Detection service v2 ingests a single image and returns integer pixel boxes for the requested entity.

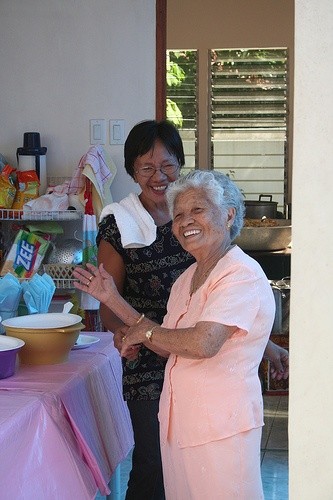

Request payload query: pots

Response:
[242,193,278,219]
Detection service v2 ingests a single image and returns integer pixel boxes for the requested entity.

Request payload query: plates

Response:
[2,313,82,329]
[72,334,101,350]
[0,335,25,351]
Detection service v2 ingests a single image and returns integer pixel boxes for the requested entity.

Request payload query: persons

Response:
[94,119,289,499]
[73,170,275,500]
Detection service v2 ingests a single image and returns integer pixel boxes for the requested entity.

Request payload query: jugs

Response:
[16,132,50,198]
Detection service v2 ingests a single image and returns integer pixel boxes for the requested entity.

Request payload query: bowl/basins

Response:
[230,219,292,252]
[0,346,25,381]
[5,323,86,367]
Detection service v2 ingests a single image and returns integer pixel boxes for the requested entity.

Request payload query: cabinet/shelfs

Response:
[1,168,106,333]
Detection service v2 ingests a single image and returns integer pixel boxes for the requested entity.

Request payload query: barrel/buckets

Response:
[268,276,291,337]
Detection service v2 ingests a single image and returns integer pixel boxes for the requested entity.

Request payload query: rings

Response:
[121,336,126,342]
[86,281,90,285]
[88,276,93,280]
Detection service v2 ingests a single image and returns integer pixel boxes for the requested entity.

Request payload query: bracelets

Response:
[136,313,144,324]
[145,326,159,345]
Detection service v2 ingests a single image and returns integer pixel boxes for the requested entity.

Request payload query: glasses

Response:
[136,163,180,175]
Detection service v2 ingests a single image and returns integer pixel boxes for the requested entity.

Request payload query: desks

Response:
[1,330,135,499]
